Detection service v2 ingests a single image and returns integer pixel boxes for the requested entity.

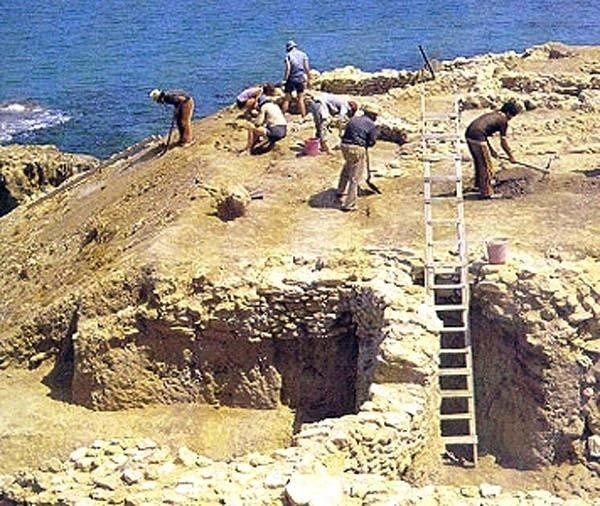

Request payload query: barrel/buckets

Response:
[486,237,508,264]
[305,137,321,156]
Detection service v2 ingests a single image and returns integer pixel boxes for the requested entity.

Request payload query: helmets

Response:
[363,103,379,114]
[347,100,357,112]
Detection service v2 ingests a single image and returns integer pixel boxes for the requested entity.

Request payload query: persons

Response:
[236,80,356,157]
[147,87,195,148]
[281,39,310,120]
[336,105,377,211]
[464,103,517,200]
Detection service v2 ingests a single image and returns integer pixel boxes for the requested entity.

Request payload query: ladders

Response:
[421,85,478,468]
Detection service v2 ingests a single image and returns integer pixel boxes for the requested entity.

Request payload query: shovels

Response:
[365,147,381,194]
[160,103,179,157]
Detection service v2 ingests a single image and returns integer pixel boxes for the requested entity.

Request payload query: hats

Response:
[257,94,268,106]
[284,40,297,52]
[149,88,164,103]
